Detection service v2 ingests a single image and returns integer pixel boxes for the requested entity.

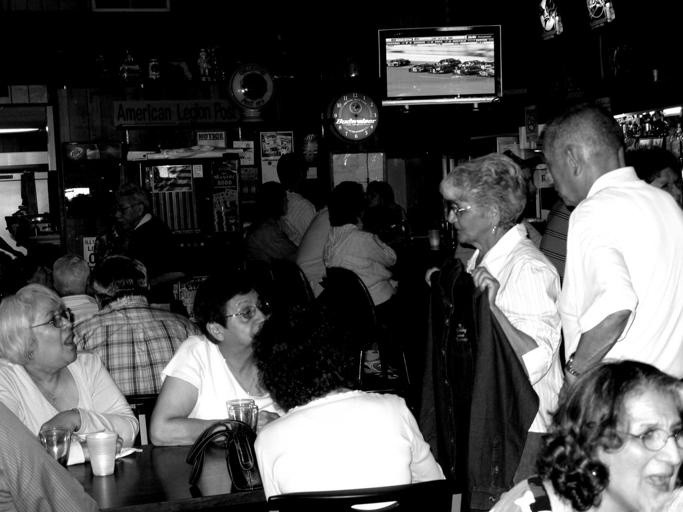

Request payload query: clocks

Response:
[329,89,382,141]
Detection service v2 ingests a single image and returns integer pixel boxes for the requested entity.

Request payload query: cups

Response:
[86,430,117,476]
[38,426,71,469]
[226,398,258,432]
[441,220,456,249]
[428,229,440,248]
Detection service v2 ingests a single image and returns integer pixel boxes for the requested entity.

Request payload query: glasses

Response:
[601,421,682,453]
[449,204,482,218]
[28,307,75,329]
[211,299,263,322]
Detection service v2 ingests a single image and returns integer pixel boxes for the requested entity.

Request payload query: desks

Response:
[50,432,267,511]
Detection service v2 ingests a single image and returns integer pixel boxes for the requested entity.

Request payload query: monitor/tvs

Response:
[377,24,504,107]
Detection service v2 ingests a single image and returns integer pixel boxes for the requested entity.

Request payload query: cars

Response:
[409,58,495,77]
[386,59,411,68]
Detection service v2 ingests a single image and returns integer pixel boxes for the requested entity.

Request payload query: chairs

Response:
[116,394,160,448]
[254,478,458,511]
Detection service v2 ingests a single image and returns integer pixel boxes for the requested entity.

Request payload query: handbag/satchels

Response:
[186,419,264,496]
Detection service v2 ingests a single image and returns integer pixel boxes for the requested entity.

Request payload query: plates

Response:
[166,144,214,156]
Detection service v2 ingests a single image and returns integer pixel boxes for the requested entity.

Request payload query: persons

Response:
[0,108,682,512]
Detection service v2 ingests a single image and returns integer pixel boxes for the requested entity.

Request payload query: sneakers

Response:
[364,359,401,381]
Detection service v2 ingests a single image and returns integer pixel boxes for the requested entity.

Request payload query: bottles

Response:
[616,109,683,158]
